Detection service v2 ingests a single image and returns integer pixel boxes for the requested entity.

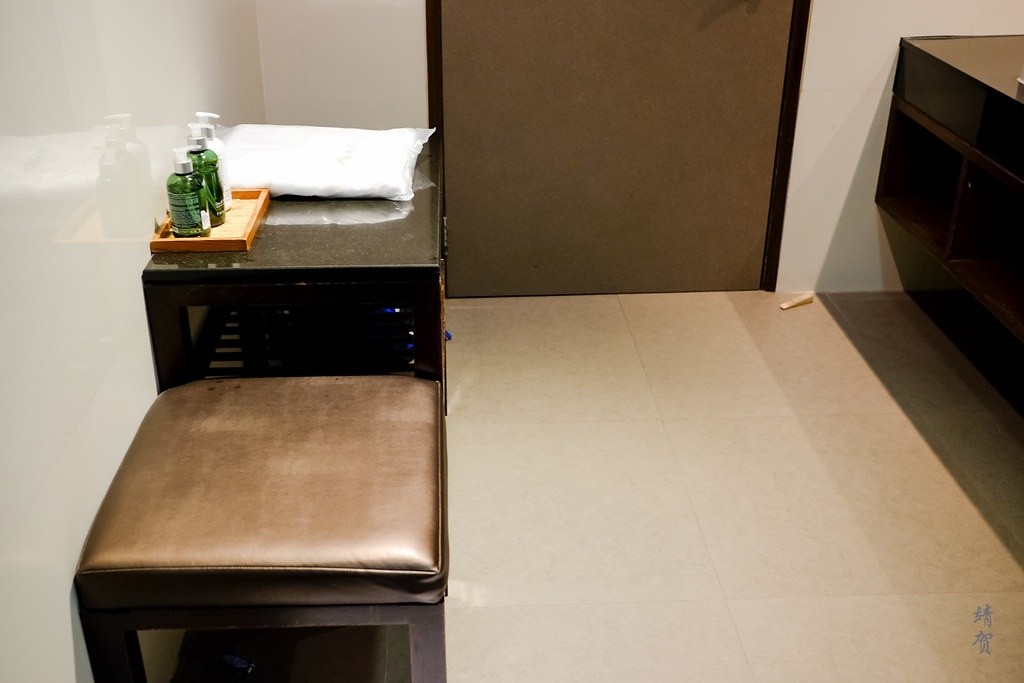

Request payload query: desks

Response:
[141,129,448,416]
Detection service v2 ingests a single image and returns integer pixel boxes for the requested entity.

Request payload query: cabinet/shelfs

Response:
[874,34,1024,420]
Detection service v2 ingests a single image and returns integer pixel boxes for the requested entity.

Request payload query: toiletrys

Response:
[165,111,233,238]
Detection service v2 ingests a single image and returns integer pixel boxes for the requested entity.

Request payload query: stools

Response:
[73,375,449,683]
